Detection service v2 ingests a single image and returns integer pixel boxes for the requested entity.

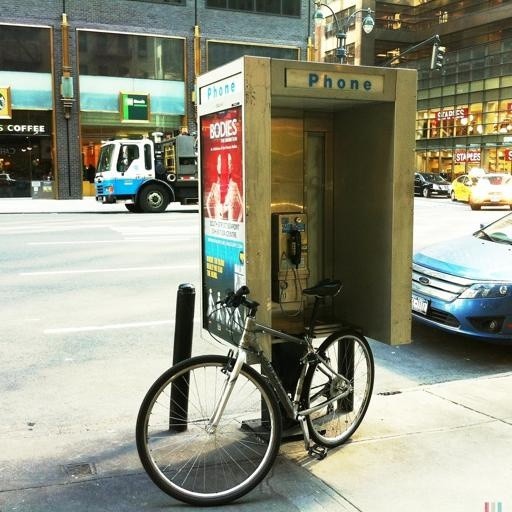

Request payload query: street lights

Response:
[313,3,376,65]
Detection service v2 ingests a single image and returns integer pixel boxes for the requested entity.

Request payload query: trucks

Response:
[92,134,197,214]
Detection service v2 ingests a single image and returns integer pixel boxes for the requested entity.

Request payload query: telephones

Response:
[271,211,311,281]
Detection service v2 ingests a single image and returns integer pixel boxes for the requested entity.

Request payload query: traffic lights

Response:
[430,43,447,73]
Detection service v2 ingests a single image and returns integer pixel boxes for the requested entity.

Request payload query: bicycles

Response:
[135,282,375,502]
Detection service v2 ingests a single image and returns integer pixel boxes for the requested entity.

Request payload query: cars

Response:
[414,166,512,213]
[410,201,512,359]
